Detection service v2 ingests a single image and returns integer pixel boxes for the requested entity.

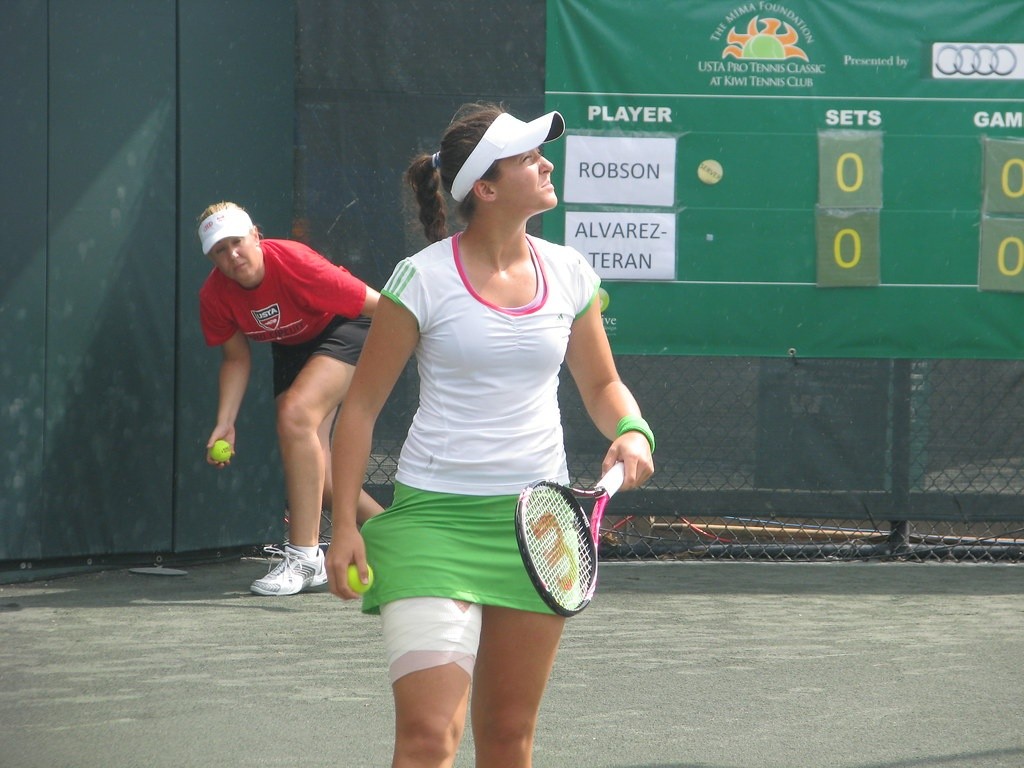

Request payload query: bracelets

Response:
[617,415,656,453]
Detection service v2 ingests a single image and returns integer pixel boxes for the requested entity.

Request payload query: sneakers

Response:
[250,543,328,595]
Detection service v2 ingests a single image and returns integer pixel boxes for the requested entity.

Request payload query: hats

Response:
[198,209,252,255]
[450,111,566,203]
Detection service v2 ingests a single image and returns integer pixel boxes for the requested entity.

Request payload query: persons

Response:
[324,104,655,768]
[198,200,387,598]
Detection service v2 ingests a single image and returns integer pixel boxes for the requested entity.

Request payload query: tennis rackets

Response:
[513,459,625,618]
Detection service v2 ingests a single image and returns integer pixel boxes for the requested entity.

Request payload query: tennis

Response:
[347,563,374,594]
[210,439,232,462]
